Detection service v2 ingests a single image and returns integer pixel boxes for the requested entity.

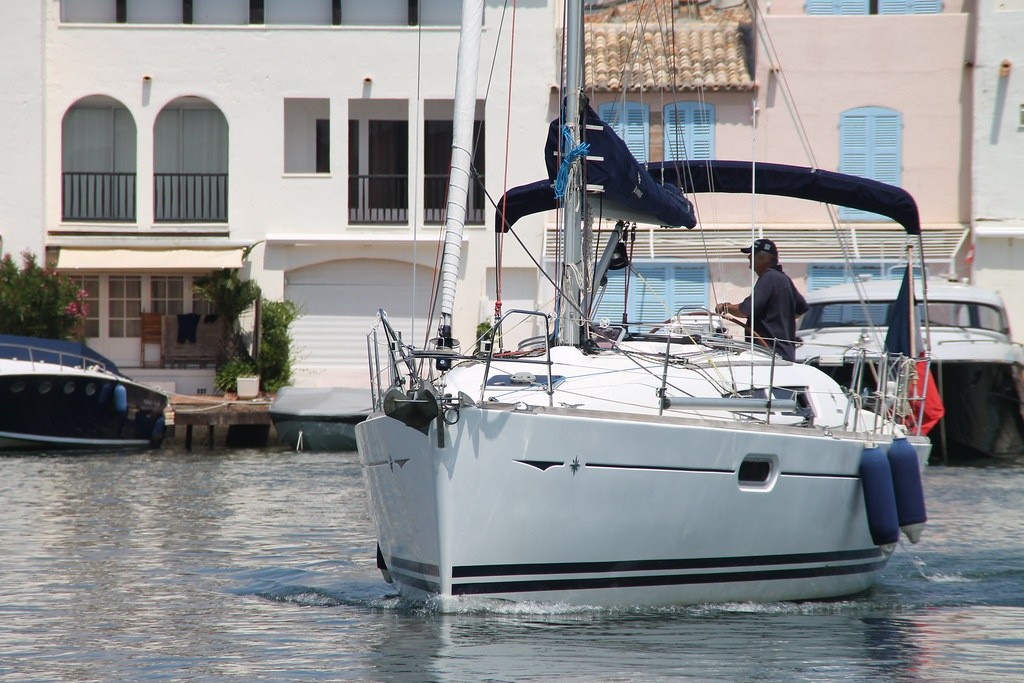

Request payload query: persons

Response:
[714,238,807,361]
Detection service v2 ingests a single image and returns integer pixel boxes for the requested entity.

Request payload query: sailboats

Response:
[353,0,944,613]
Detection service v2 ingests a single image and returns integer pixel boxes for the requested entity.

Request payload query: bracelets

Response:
[721,303,729,311]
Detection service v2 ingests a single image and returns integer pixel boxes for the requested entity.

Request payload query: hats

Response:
[741,238,778,254]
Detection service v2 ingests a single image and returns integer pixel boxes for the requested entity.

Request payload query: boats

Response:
[793,223,1024,463]
[267,385,386,452]
[0,334,168,452]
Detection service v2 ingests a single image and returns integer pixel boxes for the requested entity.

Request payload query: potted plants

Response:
[212,357,261,399]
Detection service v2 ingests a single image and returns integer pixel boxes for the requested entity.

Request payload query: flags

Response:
[884,263,943,436]
